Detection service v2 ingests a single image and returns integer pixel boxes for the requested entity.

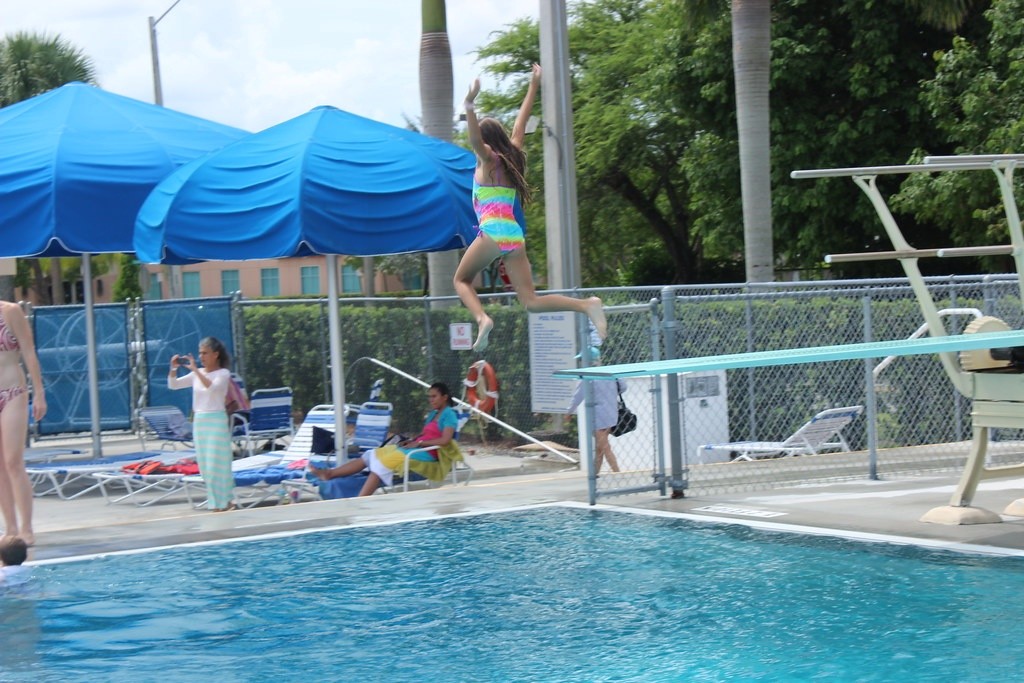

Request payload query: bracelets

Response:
[170,367,177,371]
[419,440,423,447]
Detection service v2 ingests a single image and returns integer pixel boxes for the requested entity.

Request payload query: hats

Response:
[574,346,600,359]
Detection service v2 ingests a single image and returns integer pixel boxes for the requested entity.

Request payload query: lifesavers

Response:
[462,358,501,416]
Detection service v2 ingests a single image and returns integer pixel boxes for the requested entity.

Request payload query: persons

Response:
[166,338,234,512]
[0,535,34,586]
[310,382,458,497]
[566,348,627,477]
[0,297,47,545]
[452,62,607,354]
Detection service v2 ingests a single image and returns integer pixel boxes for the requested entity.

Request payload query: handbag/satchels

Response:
[609,409,636,437]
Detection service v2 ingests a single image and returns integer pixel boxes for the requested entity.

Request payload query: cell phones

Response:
[177,358,190,365]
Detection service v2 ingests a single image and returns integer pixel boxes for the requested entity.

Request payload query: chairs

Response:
[137,406,194,450]
[91,450,286,507]
[229,387,296,456]
[280,406,475,505]
[22,447,89,483]
[695,405,864,465]
[228,402,393,512]
[181,405,350,510]
[25,452,198,501]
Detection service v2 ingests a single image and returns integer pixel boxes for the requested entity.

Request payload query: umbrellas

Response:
[0,82,528,477]
